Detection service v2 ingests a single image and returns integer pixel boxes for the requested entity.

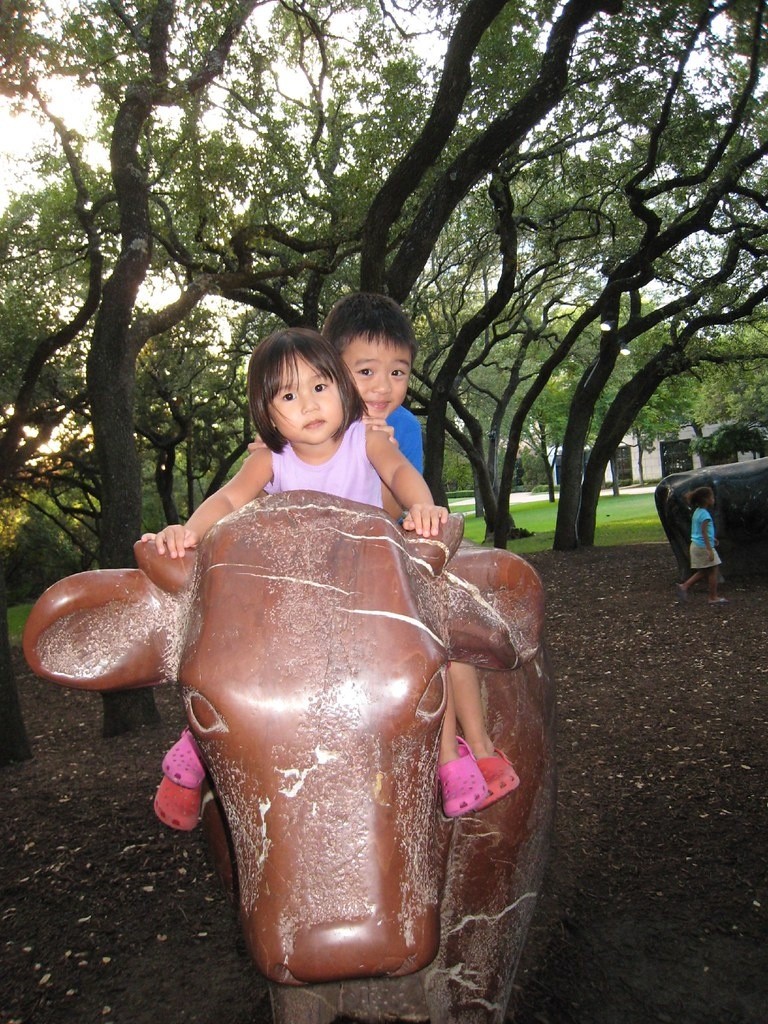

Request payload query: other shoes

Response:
[676,582,690,602]
[709,596,730,606]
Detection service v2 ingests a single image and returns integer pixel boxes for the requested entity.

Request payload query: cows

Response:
[653,455,768,592]
[21,488,560,1024]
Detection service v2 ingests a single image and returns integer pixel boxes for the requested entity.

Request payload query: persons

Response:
[154,292,519,830]
[676,487,728,605]
[141,328,486,815]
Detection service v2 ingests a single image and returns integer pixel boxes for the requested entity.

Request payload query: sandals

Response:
[162,722,207,789]
[470,746,518,812]
[154,775,202,832]
[437,734,488,817]
